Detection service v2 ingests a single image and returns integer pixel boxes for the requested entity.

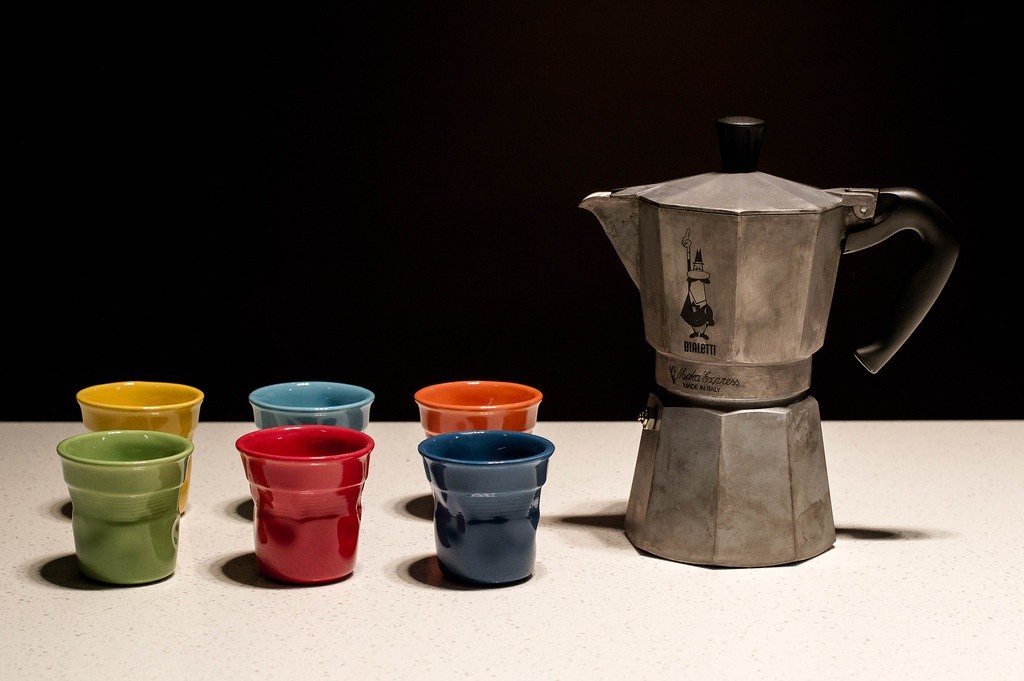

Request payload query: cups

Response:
[234,425,376,581]
[414,379,545,437]
[75,380,207,516]
[418,432,555,589]
[56,430,195,586]
[248,380,377,431]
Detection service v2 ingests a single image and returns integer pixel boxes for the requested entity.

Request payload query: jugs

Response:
[581,102,963,571]
